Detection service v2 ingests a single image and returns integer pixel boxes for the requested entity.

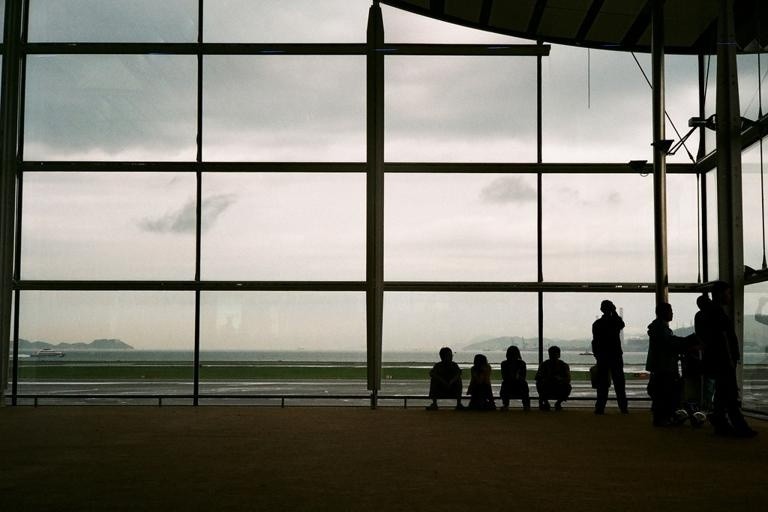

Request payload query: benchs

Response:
[4,394,653,408]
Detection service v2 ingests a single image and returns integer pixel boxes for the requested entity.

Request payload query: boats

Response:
[30,345,66,359]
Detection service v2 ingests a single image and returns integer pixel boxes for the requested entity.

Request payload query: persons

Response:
[425,348,468,412]
[466,355,498,411]
[694,295,716,410]
[591,300,629,415]
[534,347,572,410]
[645,303,679,428]
[705,282,760,441]
[500,345,532,412]
